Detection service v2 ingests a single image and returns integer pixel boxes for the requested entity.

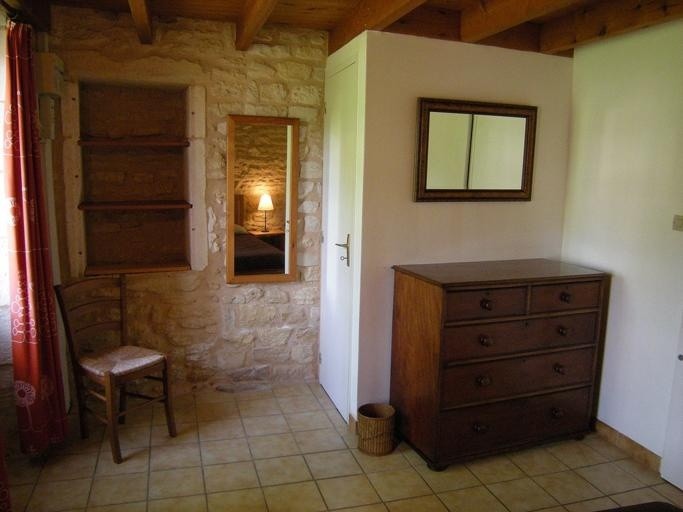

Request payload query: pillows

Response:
[233,224,250,234]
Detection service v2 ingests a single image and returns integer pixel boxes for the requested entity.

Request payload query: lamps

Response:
[256,194,274,232]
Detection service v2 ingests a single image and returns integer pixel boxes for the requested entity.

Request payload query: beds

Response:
[235,235,285,275]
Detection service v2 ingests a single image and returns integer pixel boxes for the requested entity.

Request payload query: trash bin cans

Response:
[357,403,397,456]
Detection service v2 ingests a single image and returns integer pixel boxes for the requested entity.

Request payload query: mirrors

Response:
[414,96,538,202]
[225,114,300,283]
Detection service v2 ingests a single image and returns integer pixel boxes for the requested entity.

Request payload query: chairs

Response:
[52,272,178,464]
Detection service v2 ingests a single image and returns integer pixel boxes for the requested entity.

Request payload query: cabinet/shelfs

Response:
[391,258,612,472]
[75,79,194,277]
